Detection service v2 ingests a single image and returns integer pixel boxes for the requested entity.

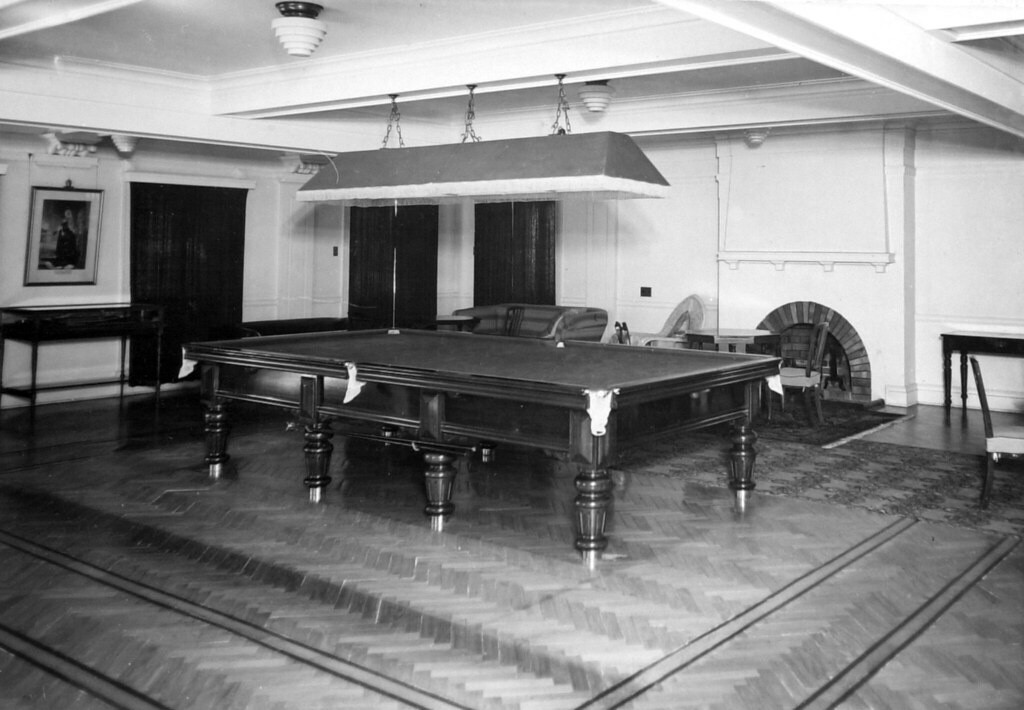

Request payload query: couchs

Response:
[452,303,609,342]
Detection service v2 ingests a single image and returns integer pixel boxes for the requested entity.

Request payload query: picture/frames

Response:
[22,185,104,286]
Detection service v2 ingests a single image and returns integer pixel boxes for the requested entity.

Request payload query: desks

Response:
[435,315,478,334]
[942,334,1024,419]
[0,304,163,441]
[177,329,783,563]
[676,333,782,408]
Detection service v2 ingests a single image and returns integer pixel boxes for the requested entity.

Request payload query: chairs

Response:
[476,304,524,335]
[765,321,832,426]
[683,334,731,417]
[971,359,1024,510]
[616,322,631,344]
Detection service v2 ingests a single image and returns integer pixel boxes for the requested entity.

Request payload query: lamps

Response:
[580,77,616,113]
[743,128,769,144]
[272,1,328,57]
[293,73,677,207]
[111,134,139,153]
